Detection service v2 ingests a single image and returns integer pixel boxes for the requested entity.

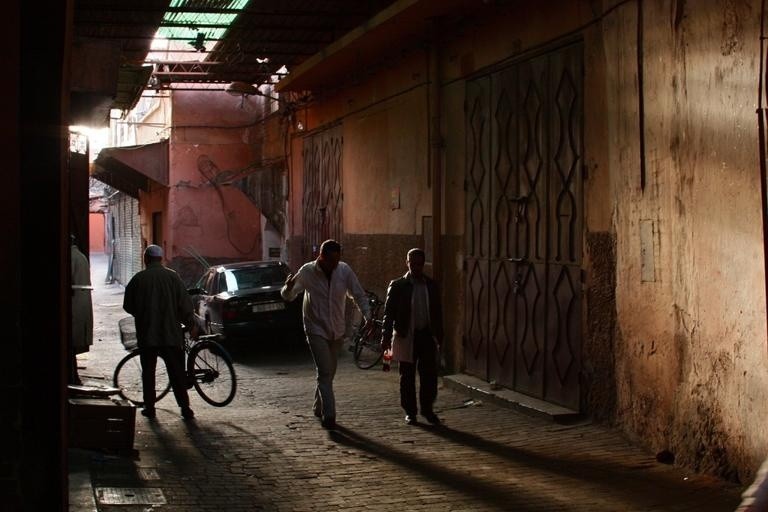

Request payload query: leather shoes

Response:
[405,411,440,424]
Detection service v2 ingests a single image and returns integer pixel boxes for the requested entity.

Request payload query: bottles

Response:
[383,349,393,372]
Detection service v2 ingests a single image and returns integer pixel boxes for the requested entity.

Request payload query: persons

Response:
[122,243,199,418]
[69,231,94,385]
[379,248,446,426]
[280,238,374,429]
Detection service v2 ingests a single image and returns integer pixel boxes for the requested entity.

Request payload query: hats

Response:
[145,245,162,256]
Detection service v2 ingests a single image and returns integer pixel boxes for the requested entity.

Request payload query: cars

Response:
[186,259,303,348]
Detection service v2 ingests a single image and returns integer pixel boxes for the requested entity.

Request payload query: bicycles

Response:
[349,289,385,370]
[113,317,237,408]
